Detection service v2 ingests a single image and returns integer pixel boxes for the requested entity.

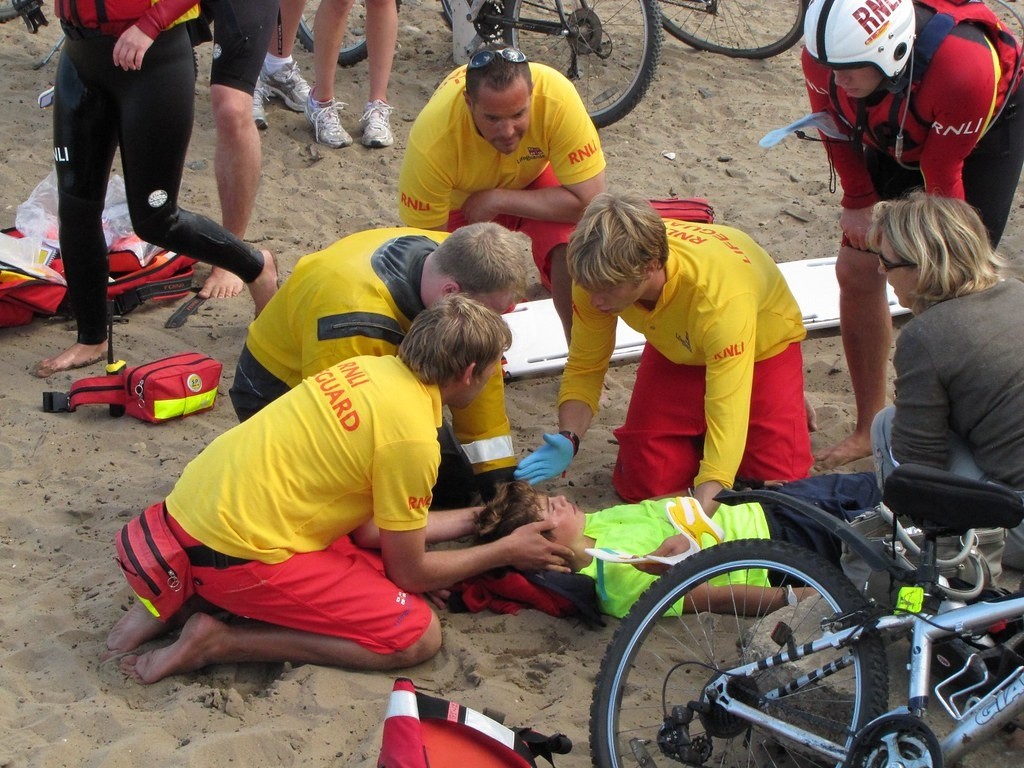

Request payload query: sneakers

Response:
[358,98,393,147]
[304,86,353,148]
[251,80,271,128]
[258,56,313,112]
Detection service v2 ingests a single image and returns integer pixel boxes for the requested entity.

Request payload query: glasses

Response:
[875,252,919,274]
[465,47,528,70]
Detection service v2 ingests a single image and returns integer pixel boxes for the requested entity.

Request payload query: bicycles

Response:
[588,461,1024,768]
[658,1,810,59]
[295,0,665,129]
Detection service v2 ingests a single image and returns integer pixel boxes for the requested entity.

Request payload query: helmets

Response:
[803,0,917,77]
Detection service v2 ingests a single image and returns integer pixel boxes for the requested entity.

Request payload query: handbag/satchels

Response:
[649,196,715,225]
[40,351,223,425]
[377,677,574,768]
[112,505,193,623]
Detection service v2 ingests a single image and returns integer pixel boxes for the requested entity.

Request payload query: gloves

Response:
[513,434,575,488]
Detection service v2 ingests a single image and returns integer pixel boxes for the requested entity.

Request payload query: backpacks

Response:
[0,216,197,328]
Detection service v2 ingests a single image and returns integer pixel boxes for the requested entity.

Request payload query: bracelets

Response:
[786,584,797,605]
[558,430,580,457]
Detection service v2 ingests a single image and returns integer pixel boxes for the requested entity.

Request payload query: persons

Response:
[228,222,542,511]
[100,295,576,687]
[474,471,883,620]
[801,0,1023,479]
[11,0,399,379]
[866,192,1024,572]
[398,44,605,349]
[513,183,816,505]
